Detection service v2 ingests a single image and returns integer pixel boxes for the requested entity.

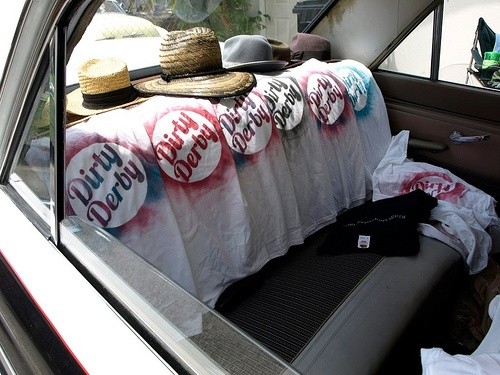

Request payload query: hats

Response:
[134,27,256,97]
[66,58,148,116]
[290,33,341,63]
[267,39,303,68]
[222,35,289,71]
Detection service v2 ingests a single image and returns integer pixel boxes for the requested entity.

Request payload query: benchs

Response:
[23,60,495,375]
